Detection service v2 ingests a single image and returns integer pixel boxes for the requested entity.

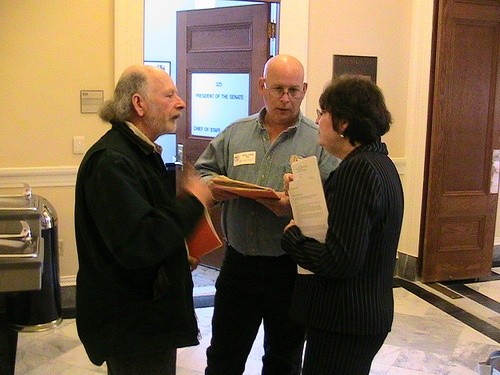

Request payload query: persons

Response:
[280,73,405,375]
[75,65,213,375]
[193,54,343,375]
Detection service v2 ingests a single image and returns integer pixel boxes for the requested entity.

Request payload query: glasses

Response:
[264,82,305,98]
[317,109,327,119]
